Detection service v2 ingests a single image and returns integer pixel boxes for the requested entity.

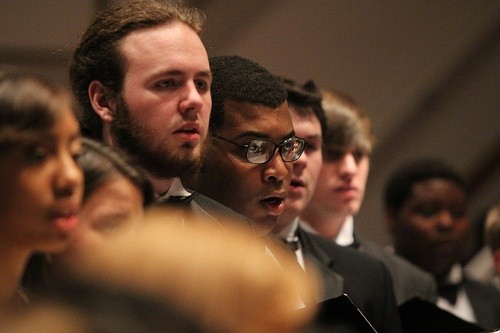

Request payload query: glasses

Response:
[212,134,307,164]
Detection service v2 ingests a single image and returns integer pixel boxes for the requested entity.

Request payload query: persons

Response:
[0,79,86,333]
[27,0,499,333]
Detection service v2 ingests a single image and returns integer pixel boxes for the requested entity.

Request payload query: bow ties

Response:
[158,191,196,209]
[282,239,299,253]
[438,281,461,306]
